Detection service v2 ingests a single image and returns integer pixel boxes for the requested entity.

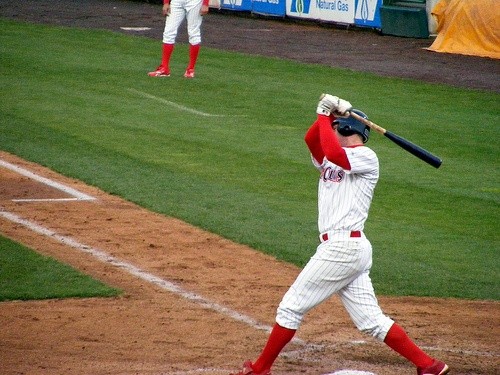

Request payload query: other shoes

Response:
[417,358,449,375]
[229,361,272,375]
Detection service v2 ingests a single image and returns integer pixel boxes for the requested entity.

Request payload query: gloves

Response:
[337,98,351,114]
[316,94,339,116]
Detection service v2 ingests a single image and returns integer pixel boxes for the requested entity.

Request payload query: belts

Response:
[323,231,361,240]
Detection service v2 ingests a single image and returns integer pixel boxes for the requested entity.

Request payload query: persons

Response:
[229,94,450,375]
[148,0,209,78]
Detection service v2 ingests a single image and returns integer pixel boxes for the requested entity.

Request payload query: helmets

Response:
[332,108,370,143]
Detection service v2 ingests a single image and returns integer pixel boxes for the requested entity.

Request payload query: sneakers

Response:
[148,65,171,76]
[184,69,195,77]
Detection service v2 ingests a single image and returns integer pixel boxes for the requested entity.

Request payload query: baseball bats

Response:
[321,92,443,169]
